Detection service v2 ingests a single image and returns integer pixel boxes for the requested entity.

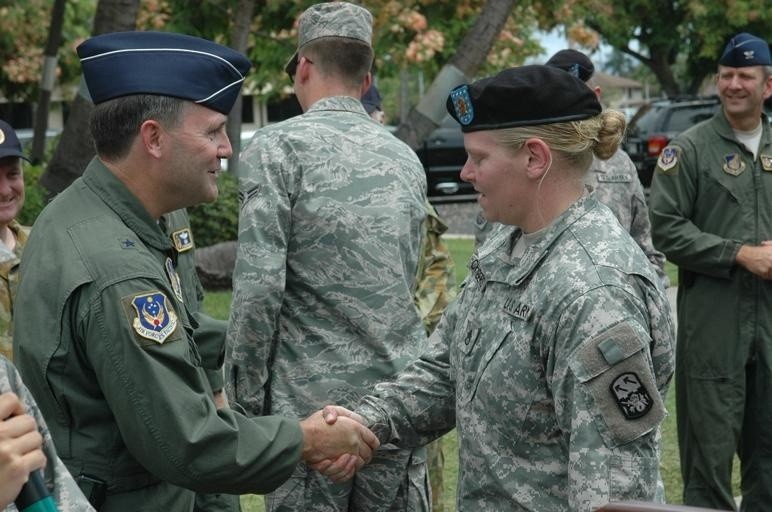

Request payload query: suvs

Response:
[608,89,701,191]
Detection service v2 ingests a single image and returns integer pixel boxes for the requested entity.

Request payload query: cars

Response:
[409,126,502,207]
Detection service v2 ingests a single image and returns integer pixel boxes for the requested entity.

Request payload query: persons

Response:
[360,81,453,512]
[647,29,771,511]
[0,118,34,356]
[222,2,432,510]
[0,348,99,512]
[308,62,675,510]
[11,31,382,510]
[469,44,669,300]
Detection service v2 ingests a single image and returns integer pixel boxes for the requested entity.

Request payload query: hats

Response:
[447,65,602,132]
[546,49,594,81]
[285,2,373,73]
[719,33,772,67]
[0,120,32,163]
[76,31,251,115]
